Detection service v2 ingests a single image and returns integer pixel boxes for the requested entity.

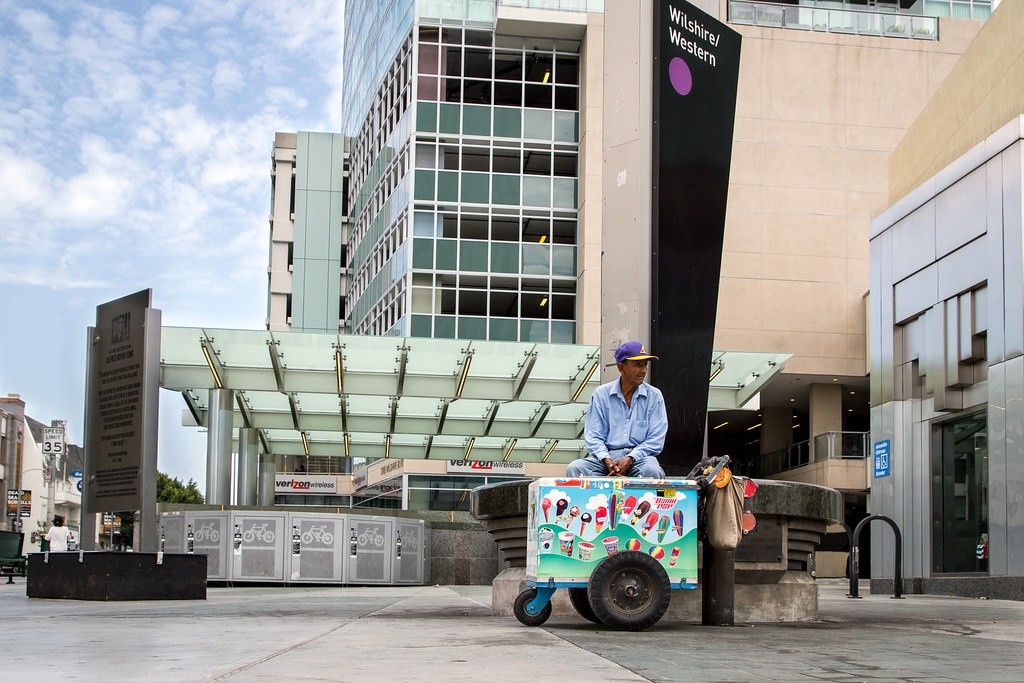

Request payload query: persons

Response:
[44,516,71,553]
[565,341,668,479]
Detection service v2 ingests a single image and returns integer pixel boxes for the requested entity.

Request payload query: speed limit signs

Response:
[42,427,65,455]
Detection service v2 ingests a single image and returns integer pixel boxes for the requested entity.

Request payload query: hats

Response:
[614,341,660,363]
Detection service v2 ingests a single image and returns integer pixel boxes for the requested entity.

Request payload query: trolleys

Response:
[510,454,732,632]
